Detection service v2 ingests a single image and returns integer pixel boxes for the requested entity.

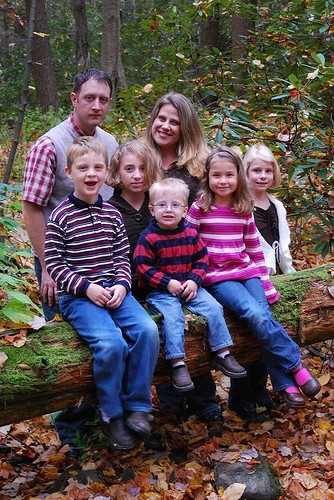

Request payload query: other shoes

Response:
[200,410,225,426]
[227,401,260,421]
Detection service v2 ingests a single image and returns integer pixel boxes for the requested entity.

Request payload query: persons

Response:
[185,147,321,407]
[22,68,299,457]
[133,178,249,393]
[45,135,160,450]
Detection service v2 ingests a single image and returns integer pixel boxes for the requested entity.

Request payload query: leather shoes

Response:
[293,362,321,397]
[99,415,140,451]
[169,362,195,392]
[276,389,306,406]
[125,411,153,435]
[208,354,248,378]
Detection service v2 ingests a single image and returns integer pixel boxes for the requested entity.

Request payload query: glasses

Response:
[153,202,186,210]
